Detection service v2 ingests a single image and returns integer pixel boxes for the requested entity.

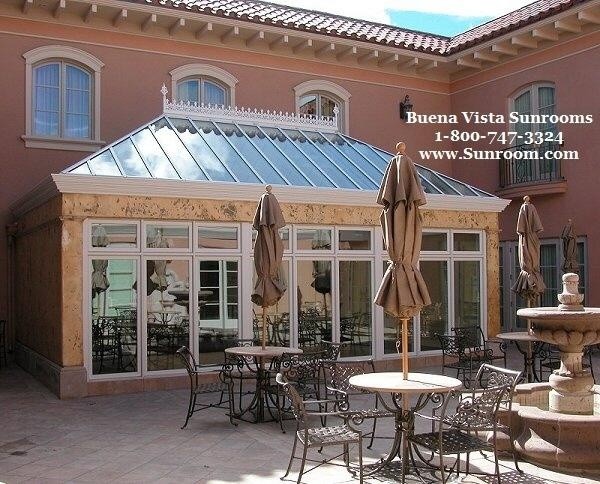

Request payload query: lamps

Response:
[399,95,414,121]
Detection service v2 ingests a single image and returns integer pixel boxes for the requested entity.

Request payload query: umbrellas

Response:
[249,185,286,418]
[148,228,172,309]
[91,223,110,316]
[311,230,332,316]
[371,140,427,474]
[561,218,580,273]
[511,195,543,383]
[298,286,303,312]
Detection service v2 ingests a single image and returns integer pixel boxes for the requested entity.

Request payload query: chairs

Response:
[1,318,8,370]
